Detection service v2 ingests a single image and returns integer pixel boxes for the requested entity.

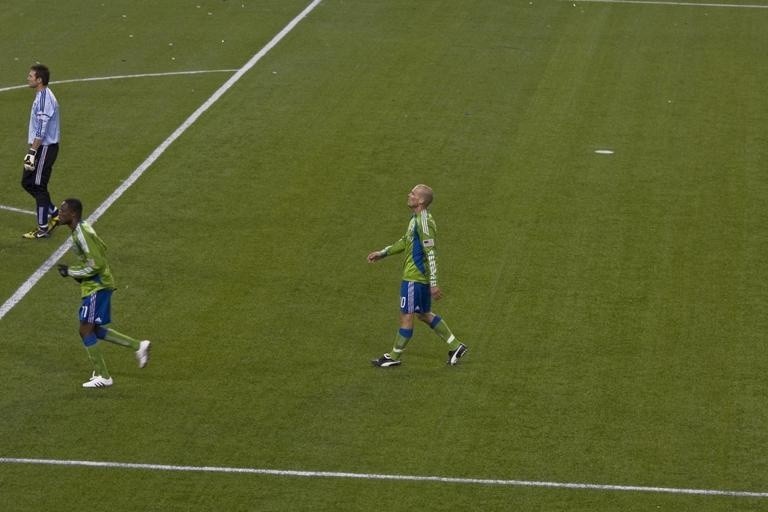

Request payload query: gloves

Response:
[23,148,37,171]
[56,263,68,277]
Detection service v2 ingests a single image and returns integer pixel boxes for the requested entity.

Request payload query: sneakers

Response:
[369,352,402,368]
[446,343,469,368]
[81,374,115,388]
[47,215,60,233]
[135,339,153,368]
[22,225,49,239]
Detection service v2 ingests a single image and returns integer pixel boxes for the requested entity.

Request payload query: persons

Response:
[20,65,63,240]
[55,199,151,387]
[365,184,468,370]
